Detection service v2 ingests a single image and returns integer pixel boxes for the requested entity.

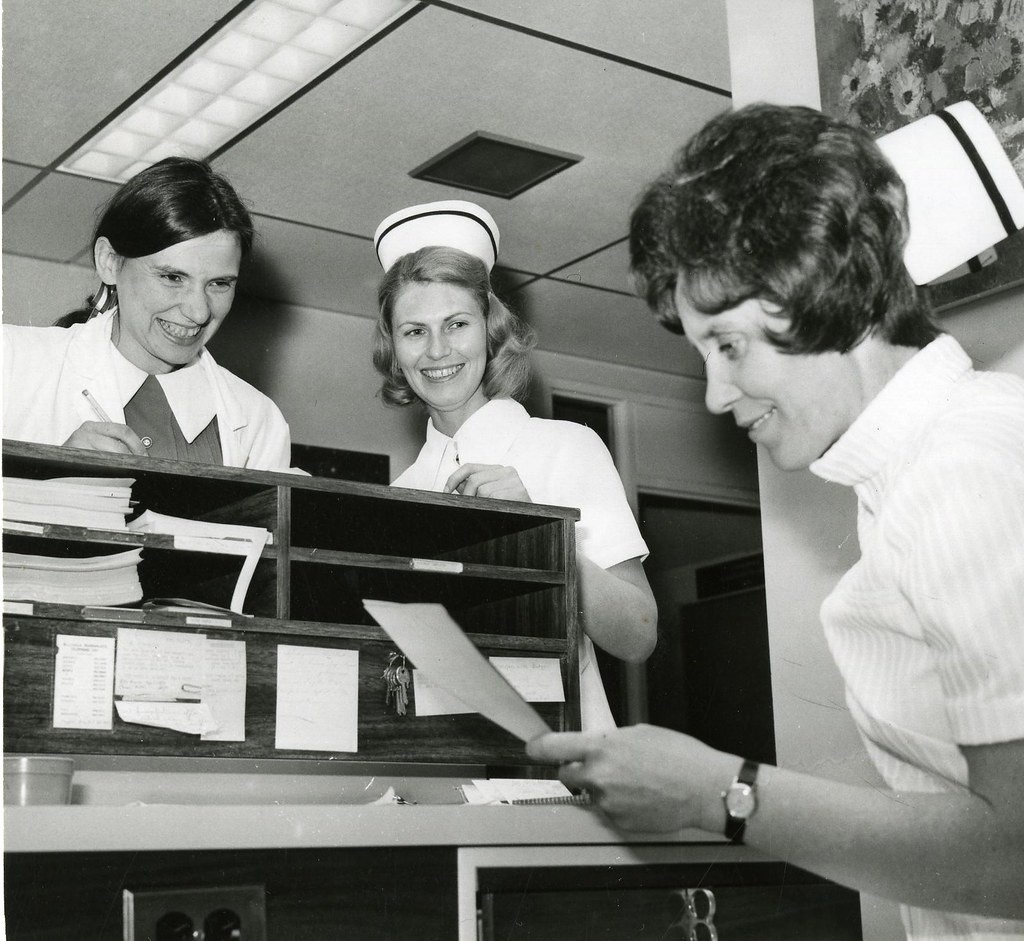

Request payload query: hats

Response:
[872,100,1023,287]
[373,200,502,274]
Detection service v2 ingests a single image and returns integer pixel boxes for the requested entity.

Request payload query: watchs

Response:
[721,755,761,847]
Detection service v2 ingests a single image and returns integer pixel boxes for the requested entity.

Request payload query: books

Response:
[2,478,274,618]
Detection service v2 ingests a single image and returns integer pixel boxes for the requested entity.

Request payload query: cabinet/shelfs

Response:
[2,436,582,780]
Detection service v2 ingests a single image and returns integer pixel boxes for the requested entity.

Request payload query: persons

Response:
[2,155,291,471]
[526,101,1024,941]
[372,200,659,734]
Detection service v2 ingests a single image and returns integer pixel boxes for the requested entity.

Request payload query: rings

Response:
[581,789,586,796]
